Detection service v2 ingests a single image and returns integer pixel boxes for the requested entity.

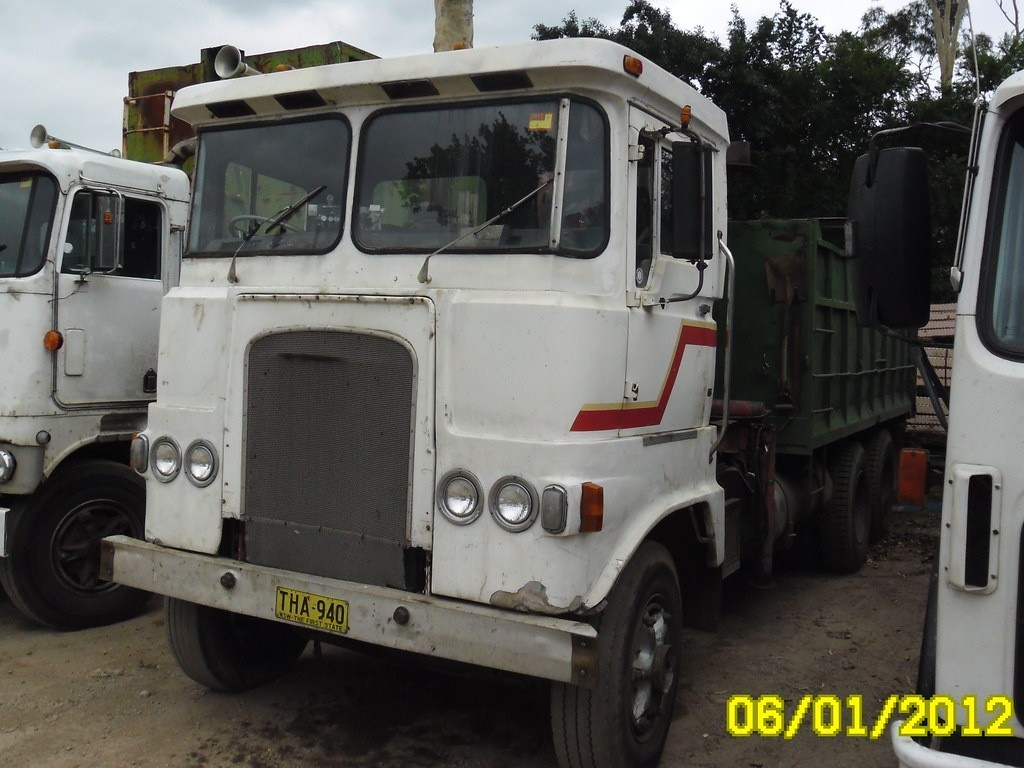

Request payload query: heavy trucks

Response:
[99,37,919,767]
[851,67,1023,768]
[0,124,192,629]
